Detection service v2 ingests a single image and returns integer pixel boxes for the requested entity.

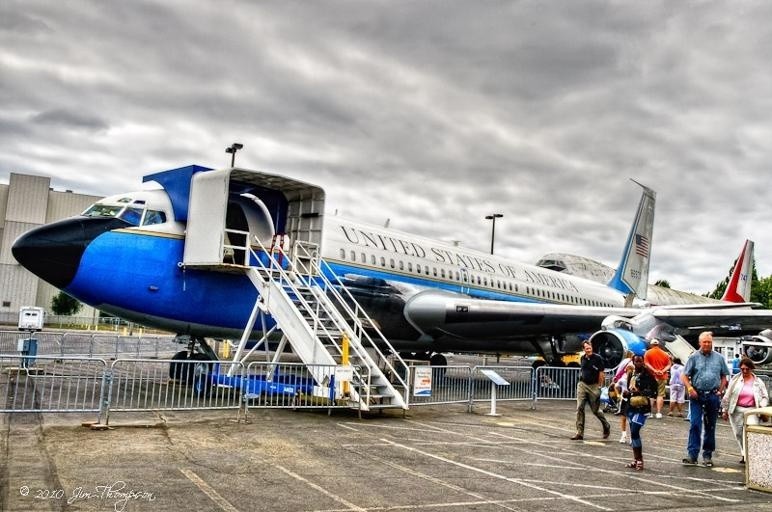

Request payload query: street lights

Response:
[484,213,502,254]
[10,162,772,384]
[225,142,244,166]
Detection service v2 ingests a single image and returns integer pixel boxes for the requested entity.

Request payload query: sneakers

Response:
[739,456,746,464]
[648,411,684,419]
[620,435,633,446]
[602,426,610,439]
[569,434,583,440]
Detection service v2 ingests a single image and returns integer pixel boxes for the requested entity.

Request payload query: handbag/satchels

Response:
[630,396,648,408]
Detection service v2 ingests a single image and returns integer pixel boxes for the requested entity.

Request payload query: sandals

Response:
[681,457,699,467]
[636,461,644,470]
[701,458,714,467]
[624,461,637,468]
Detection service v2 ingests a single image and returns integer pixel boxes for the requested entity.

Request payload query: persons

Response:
[570,339,611,440]
[600,380,613,410]
[667,358,685,417]
[679,330,730,467]
[719,357,769,465]
[612,338,672,471]
[741,354,746,359]
[731,354,741,378]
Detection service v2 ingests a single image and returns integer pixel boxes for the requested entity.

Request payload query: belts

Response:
[696,389,717,395]
[736,405,754,409]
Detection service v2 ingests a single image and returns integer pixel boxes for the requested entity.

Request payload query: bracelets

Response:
[687,386,694,394]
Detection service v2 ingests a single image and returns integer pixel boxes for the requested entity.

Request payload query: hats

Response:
[650,338,659,345]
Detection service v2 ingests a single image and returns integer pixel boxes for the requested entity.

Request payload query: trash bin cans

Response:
[744,406,772,492]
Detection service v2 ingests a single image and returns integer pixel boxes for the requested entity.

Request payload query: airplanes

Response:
[402,239,772,389]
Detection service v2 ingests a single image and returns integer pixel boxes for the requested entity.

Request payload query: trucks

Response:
[18,304,44,332]
